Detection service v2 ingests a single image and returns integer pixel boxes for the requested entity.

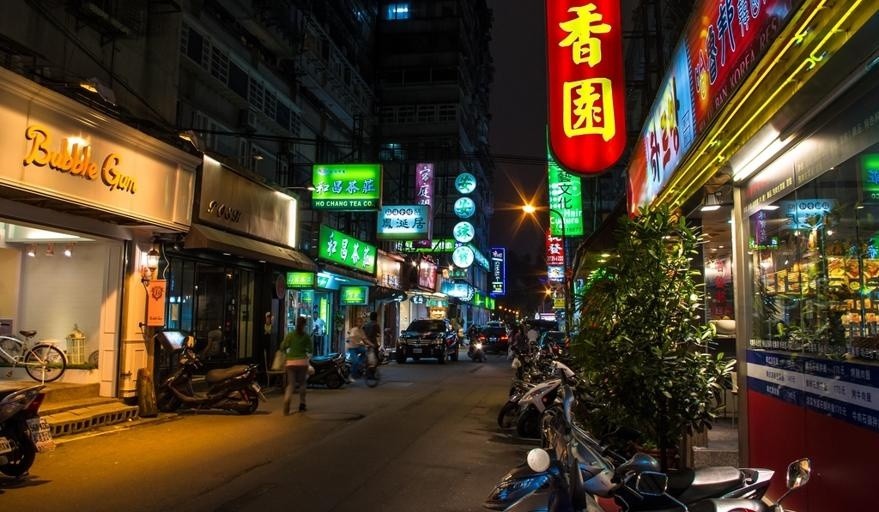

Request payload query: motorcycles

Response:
[635,457,811,512]
[483,361,777,511]
[465,333,488,363]
[482,340,583,434]
[297,333,351,392]
[158,332,266,416]
[0,382,53,482]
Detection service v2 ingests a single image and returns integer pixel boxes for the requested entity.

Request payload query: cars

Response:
[508,319,559,343]
[538,331,569,350]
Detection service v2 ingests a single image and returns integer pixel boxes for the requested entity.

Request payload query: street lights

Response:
[519,204,571,332]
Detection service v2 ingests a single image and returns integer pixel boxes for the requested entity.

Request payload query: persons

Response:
[347,317,375,384]
[362,312,381,353]
[312,311,326,357]
[450,314,539,353]
[203,323,222,362]
[280,317,312,416]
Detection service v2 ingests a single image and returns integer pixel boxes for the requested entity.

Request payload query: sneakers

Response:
[297,403,311,415]
[278,399,293,417]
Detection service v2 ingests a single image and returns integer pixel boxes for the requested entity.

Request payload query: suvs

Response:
[479,325,510,357]
[395,317,461,364]
[486,320,509,333]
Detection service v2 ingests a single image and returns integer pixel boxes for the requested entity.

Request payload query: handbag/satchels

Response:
[264,348,292,375]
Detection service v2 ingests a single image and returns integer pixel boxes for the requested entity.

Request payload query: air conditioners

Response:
[240,105,259,131]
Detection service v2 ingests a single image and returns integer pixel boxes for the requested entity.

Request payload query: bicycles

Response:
[335,331,392,389]
[0,329,71,388]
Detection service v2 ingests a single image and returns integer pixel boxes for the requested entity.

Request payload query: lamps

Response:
[284,182,316,193]
[177,128,206,154]
[146,239,161,278]
[699,192,734,214]
[234,150,264,164]
[79,74,118,107]
[26,241,77,260]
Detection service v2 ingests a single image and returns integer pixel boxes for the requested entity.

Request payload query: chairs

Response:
[709,318,736,350]
[722,361,739,429]
